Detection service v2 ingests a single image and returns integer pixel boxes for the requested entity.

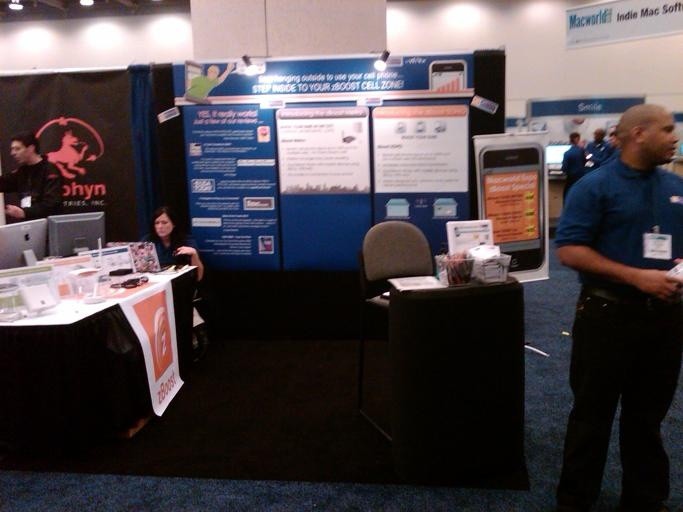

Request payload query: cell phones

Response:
[478,140,544,272]
[429,60,467,92]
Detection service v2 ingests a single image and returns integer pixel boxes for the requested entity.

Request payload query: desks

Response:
[387,271,531,478]
[0,265,201,446]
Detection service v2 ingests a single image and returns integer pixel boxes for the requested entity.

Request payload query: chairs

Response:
[343,220,434,413]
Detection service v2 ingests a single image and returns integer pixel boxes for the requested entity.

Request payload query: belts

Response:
[579,291,664,312]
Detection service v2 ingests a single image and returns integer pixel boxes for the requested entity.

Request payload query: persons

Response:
[550,103,682,512]
[552,121,626,214]
[0,131,66,233]
[140,208,205,314]
[182,63,237,102]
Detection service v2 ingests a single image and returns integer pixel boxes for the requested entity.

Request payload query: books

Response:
[448,220,500,283]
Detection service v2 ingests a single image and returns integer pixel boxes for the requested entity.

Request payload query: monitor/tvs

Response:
[48,211,106,259]
[0,219,52,268]
